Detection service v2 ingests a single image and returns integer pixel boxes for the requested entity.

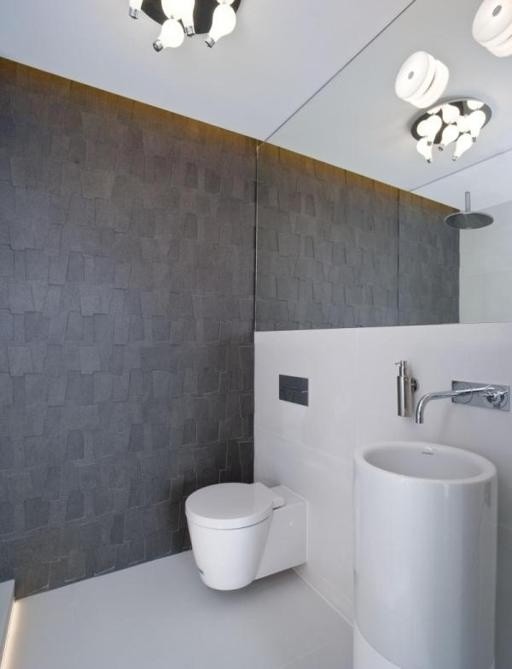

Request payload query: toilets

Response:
[185,481,310,594]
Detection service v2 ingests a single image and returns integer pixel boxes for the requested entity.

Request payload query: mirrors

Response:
[253,0,511,330]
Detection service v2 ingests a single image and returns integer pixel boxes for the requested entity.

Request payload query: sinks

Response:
[350,442,498,667]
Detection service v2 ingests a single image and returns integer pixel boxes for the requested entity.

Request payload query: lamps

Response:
[472,0,512,57]
[129,0,240,53]
[394,51,450,109]
[407,96,492,163]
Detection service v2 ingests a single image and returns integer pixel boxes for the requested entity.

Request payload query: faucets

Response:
[412,383,509,426]
